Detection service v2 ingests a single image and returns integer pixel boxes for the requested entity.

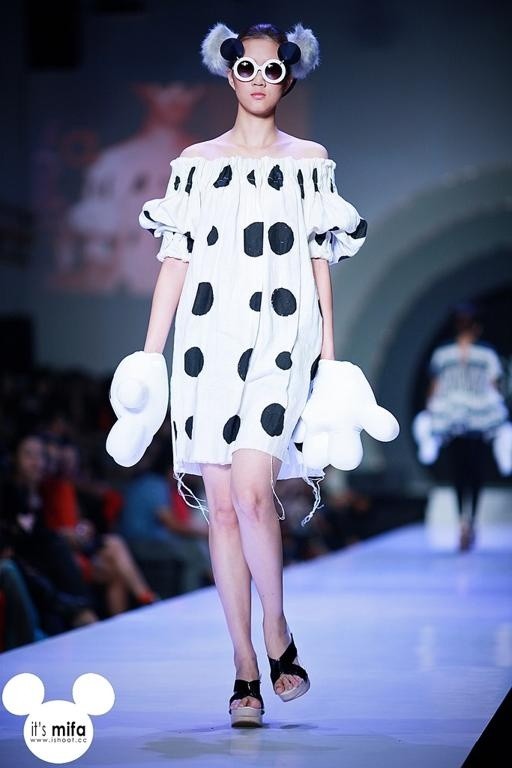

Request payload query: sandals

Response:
[227,673,265,729]
[267,632,311,703]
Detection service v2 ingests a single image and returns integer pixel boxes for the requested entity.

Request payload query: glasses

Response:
[217,38,301,82]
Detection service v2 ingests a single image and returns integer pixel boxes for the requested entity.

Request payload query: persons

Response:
[104,20,404,730]
[0,362,372,649]
[413,300,509,552]
